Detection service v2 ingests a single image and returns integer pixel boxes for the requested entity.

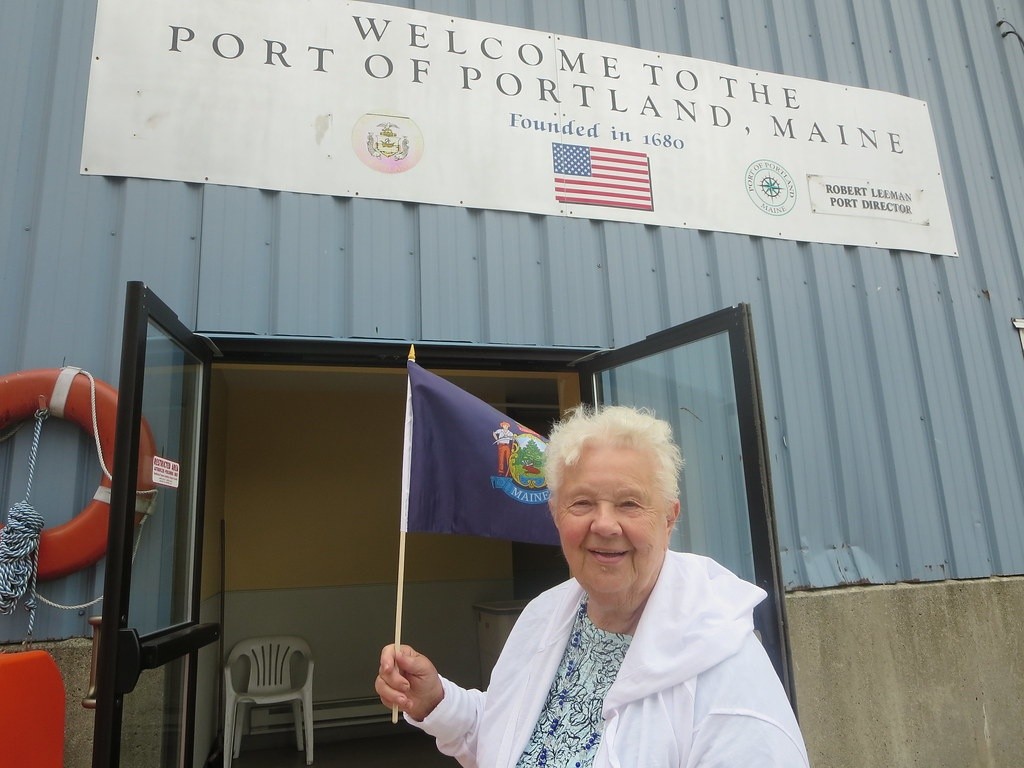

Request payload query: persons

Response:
[374,404,811,768]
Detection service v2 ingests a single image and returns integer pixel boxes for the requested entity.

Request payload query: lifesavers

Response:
[0,366,157,584]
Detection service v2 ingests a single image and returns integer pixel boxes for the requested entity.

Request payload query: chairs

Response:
[224,635,314,768]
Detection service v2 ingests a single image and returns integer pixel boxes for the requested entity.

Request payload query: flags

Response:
[406,361,563,546]
